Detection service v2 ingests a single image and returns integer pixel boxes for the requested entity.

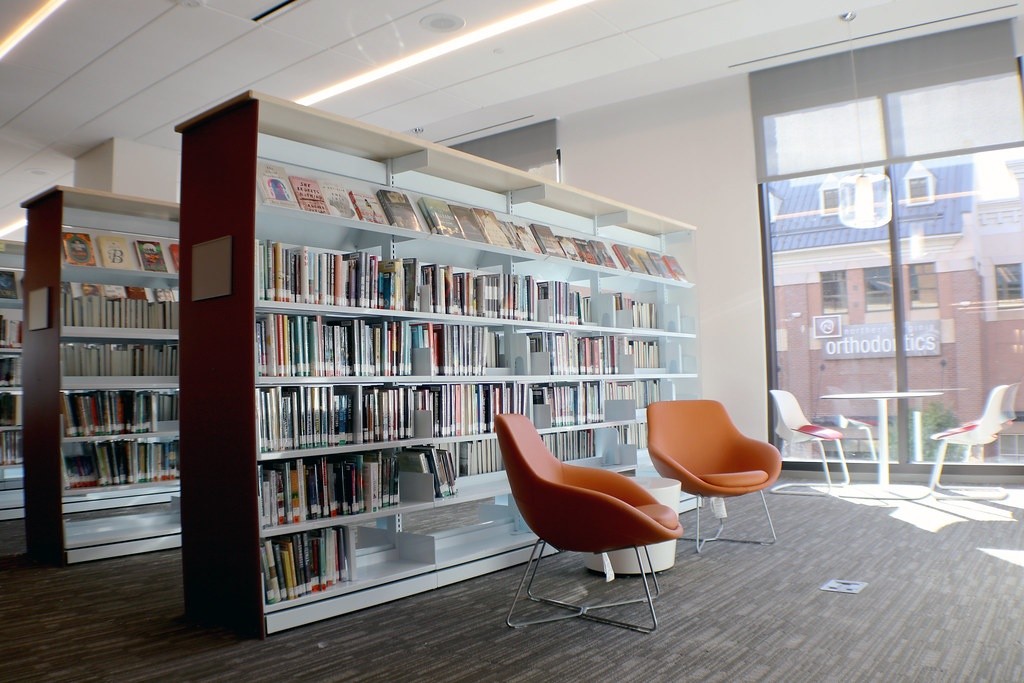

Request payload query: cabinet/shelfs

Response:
[175,89,696,642]
[0,238,29,523]
[17,185,181,568]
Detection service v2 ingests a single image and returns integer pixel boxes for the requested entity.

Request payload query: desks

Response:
[583,476,682,575]
[816,390,944,504]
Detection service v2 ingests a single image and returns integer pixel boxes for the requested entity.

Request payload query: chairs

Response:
[769,389,851,496]
[648,400,783,554]
[493,411,684,635]
[930,385,1010,504]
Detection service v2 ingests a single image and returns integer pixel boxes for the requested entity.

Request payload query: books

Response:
[647,251,674,280]
[555,235,586,263]
[571,237,600,265]
[662,255,690,283]
[514,224,543,254]
[631,246,660,277]
[60,226,181,490]
[348,189,390,225]
[0,269,24,467]
[253,239,659,605]
[470,207,512,250]
[587,240,617,270]
[417,196,464,238]
[376,189,423,232]
[288,175,330,214]
[448,204,488,244]
[257,161,300,209]
[317,180,359,221]
[498,220,526,252]
[528,223,567,258]
[612,244,648,275]
